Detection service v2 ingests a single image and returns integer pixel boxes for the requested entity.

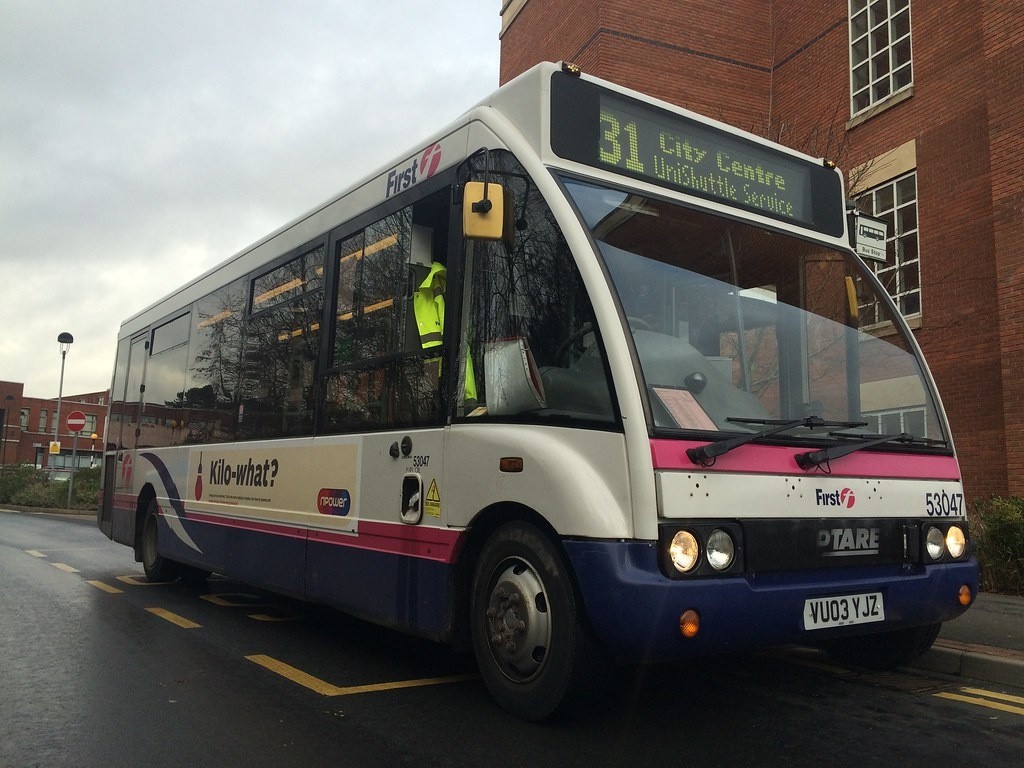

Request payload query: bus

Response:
[96,61,984,725]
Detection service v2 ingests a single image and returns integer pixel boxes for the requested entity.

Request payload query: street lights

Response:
[2,395,15,470]
[90,434,98,467]
[51,332,74,479]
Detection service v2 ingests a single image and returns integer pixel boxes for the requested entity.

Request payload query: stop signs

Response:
[66,411,86,432]
